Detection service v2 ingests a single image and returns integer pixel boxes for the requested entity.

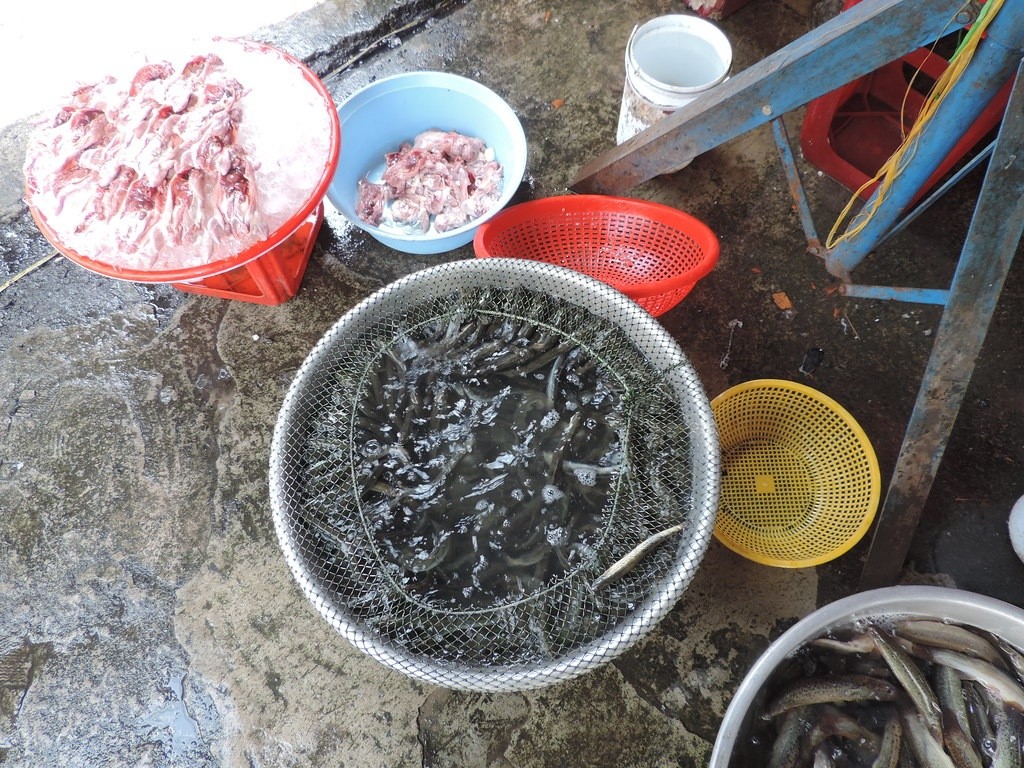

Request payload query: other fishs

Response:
[299,285,692,670]
[737,611,1023,767]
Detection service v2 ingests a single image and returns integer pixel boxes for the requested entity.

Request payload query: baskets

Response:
[709,378,883,570]
[473,193,719,319]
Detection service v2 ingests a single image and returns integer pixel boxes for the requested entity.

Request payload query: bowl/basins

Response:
[27,33,340,283]
[324,72,528,255]
[713,586,1024,768]
[269,258,721,693]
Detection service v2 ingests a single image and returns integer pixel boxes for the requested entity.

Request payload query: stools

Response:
[796,0,1024,219]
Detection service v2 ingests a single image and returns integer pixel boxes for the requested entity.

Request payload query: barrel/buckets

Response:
[616,15,732,173]
